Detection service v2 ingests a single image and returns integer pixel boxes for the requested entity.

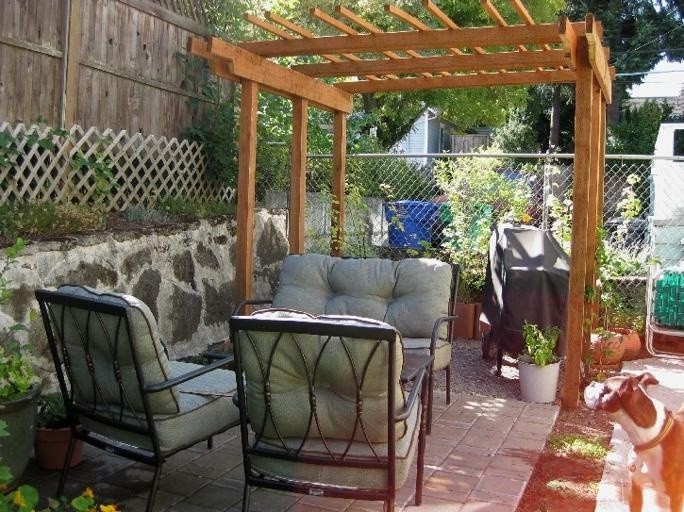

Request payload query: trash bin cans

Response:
[385,200,493,256]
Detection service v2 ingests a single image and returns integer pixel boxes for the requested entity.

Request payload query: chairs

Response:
[36,286,248,512]
[228,307,426,511]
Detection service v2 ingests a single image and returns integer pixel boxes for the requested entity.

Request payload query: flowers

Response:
[36,487,117,511]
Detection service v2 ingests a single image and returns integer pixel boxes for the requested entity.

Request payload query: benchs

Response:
[228,252,463,435]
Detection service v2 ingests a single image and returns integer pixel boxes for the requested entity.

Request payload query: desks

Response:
[201,340,435,435]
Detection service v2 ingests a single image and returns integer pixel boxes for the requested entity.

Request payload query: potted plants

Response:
[36,391,83,470]
[1,234,41,497]
[582,223,661,369]
[515,316,563,405]
[448,268,490,341]
